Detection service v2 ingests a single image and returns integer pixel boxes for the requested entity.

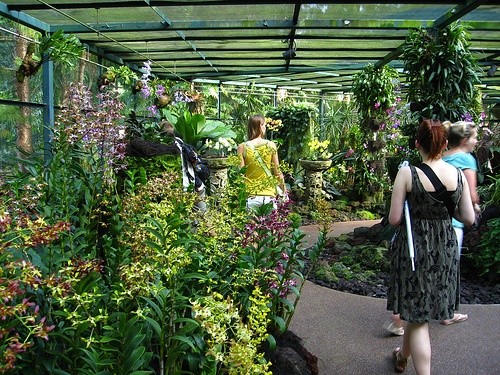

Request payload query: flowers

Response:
[308,137,330,153]
[264,116,284,132]
[204,136,237,151]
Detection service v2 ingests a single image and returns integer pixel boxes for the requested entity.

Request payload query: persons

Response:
[238,115,287,215]
[387,121,479,336]
[387,120,475,375]
[161,122,207,212]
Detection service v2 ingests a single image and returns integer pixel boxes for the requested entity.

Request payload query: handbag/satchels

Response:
[270,177,290,204]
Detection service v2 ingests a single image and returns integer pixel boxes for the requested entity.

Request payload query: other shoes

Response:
[393,347,408,373]
[441,313,469,325]
[380,320,405,335]
